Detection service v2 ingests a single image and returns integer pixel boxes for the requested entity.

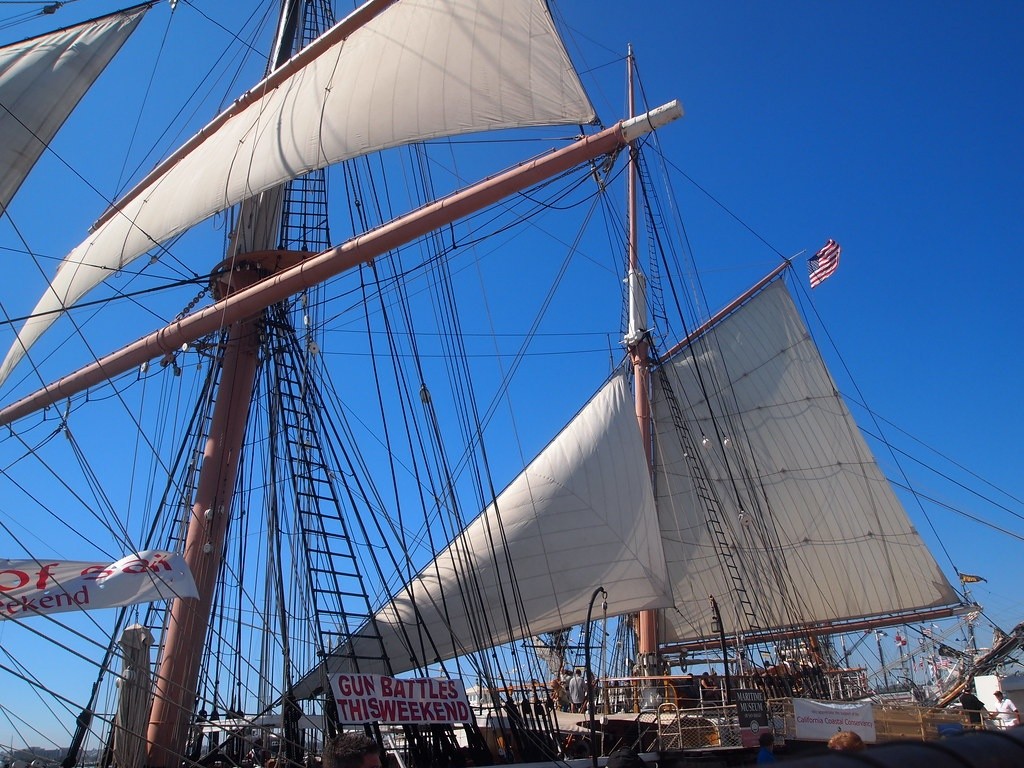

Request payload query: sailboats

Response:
[1,0,1023,768]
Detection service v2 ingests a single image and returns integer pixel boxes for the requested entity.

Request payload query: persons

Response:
[254,749,272,768]
[960,686,992,730]
[569,669,587,713]
[549,666,574,713]
[756,733,777,768]
[827,730,868,752]
[604,748,647,768]
[700,672,722,706]
[322,732,383,768]
[989,691,1021,728]
[751,654,820,698]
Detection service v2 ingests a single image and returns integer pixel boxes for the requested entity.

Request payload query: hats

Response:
[965,683,970,691]
[993,691,1002,695]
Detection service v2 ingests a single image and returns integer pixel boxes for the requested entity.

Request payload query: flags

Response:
[961,573,988,584]
[806,238,841,290]
[894,626,952,676]
